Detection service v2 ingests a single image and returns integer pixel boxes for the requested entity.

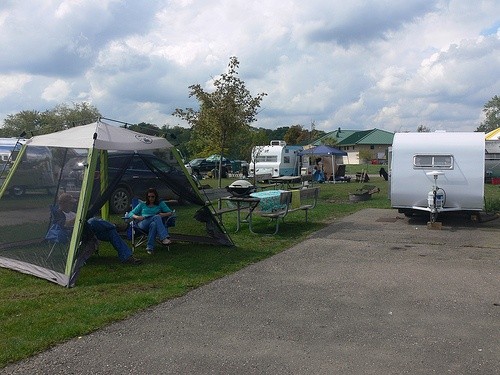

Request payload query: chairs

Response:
[123,197,177,253]
[44,205,98,266]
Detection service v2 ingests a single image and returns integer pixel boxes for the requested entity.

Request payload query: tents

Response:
[0,120,235,287]
[296,145,348,184]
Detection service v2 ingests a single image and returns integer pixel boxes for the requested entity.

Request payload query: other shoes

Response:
[120,256,143,264]
[162,239,171,245]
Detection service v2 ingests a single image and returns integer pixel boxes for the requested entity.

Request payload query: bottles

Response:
[125,212,129,222]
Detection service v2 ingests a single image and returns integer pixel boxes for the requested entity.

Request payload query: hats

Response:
[59,193,77,203]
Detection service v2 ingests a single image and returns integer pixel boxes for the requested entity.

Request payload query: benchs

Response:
[203,188,256,232]
[262,187,320,233]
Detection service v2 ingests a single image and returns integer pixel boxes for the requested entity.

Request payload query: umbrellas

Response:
[206,154,227,177]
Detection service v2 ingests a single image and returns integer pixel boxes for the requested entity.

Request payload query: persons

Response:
[314,157,324,183]
[128,189,172,256]
[53,193,144,265]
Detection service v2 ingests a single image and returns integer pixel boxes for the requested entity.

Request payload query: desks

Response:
[223,190,291,234]
[273,176,301,189]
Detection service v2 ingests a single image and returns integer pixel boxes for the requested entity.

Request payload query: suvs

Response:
[184,158,228,179]
[56,152,198,215]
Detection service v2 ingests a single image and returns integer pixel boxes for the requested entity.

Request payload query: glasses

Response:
[147,196,155,198]
